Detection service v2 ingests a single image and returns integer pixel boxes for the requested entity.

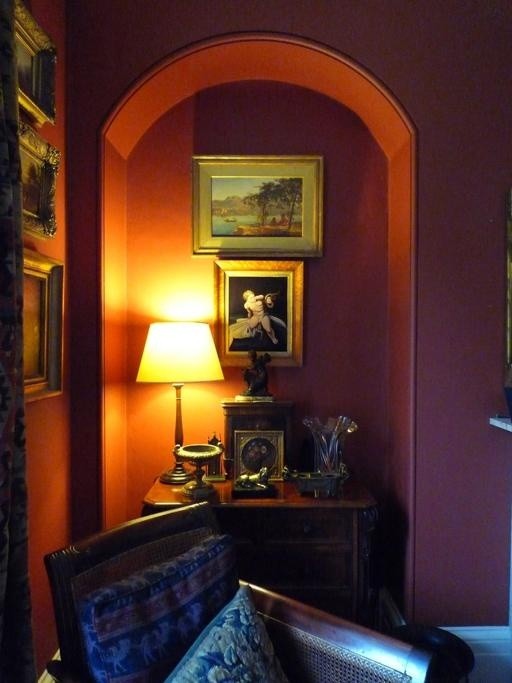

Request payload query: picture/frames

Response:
[14,0,59,130]
[213,260,305,368]
[234,430,284,482]
[23,245,66,408]
[18,121,62,243]
[192,154,324,259]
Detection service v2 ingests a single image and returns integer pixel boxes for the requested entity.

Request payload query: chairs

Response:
[43,499,434,683]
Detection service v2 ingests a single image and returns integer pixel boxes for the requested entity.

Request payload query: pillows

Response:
[78,531,240,682]
[164,585,289,682]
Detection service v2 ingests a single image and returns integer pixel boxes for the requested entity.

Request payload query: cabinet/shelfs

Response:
[143,475,376,622]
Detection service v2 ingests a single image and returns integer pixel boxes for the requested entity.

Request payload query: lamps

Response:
[136,322,225,485]
